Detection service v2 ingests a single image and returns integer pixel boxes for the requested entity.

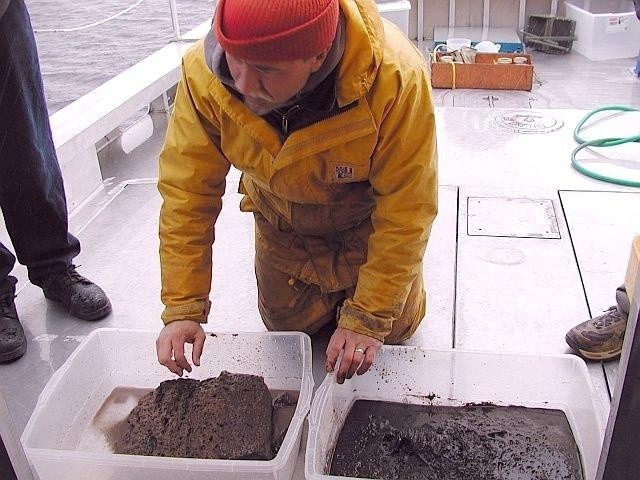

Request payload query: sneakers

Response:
[565,286,631,360]
[43,266,112,319]
[0,299,27,362]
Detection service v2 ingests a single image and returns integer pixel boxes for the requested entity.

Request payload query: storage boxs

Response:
[18,329,317,478]
[564,0,639,60]
[304,343,604,479]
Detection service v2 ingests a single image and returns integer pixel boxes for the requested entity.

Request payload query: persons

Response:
[156,0,438,384]
[0,0,112,362]
[566,0,640,362]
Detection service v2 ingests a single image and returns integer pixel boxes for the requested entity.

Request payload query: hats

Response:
[215,0,339,60]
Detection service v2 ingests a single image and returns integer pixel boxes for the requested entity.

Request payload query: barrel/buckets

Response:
[474,40,502,53]
[446,37,471,54]
